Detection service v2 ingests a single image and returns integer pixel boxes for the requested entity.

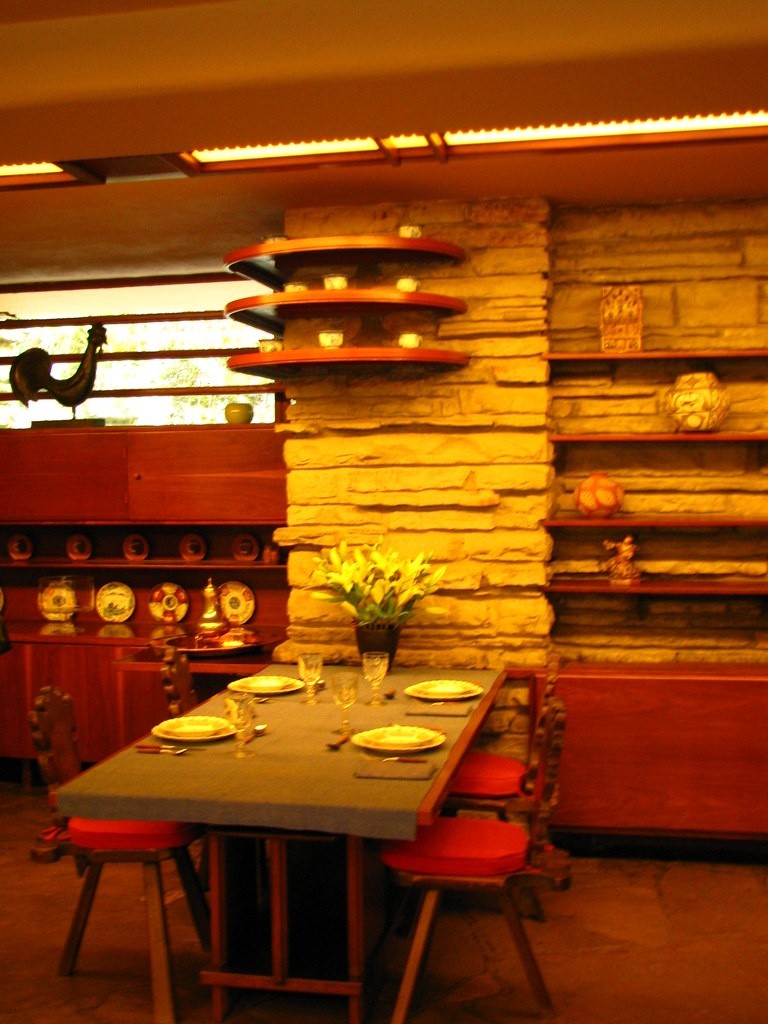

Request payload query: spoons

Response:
[244,724,269,743]
[137,748,189,755]
[328,734,349,749]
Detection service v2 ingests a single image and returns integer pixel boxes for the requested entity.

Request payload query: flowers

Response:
[309,543,448,621]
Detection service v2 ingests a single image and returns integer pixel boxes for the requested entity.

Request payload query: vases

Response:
[357,622,402,676]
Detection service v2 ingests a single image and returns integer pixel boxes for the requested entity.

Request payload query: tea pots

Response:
[196,578,228,639]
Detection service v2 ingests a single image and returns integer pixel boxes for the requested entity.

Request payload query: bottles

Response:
[573,472,625,517]
[663,372,731,432]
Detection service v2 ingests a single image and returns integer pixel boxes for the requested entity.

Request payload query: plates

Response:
[151,627,282,655]
[227,675,305,695]
[350,727,445,753]
[403,679,483,701]
[152,716,237,740]
[6,533,260,562]
[39,582,255,625]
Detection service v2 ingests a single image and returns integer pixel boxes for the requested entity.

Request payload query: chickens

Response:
[10,321,110,412]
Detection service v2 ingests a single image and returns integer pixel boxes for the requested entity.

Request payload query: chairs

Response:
[28,683,210,1024]
[379,652,570,1024]
[158,643,196,716]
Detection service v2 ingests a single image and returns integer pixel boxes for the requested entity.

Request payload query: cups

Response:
[282,273,417,292]
[259,330,420,353]
[400,225,421,237]
[225,404,254,423]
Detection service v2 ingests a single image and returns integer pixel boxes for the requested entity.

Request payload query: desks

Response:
[52,664,509,1024]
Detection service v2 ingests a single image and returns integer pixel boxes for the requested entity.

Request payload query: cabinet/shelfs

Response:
[540,349,768,658]
[0,424,287,761]
[222,236,469,372]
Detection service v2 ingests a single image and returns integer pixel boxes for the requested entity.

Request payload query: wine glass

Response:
[296,651,390,735]
[224,693,256,759]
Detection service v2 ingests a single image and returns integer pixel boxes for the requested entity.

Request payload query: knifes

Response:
[134,743,207,751]
